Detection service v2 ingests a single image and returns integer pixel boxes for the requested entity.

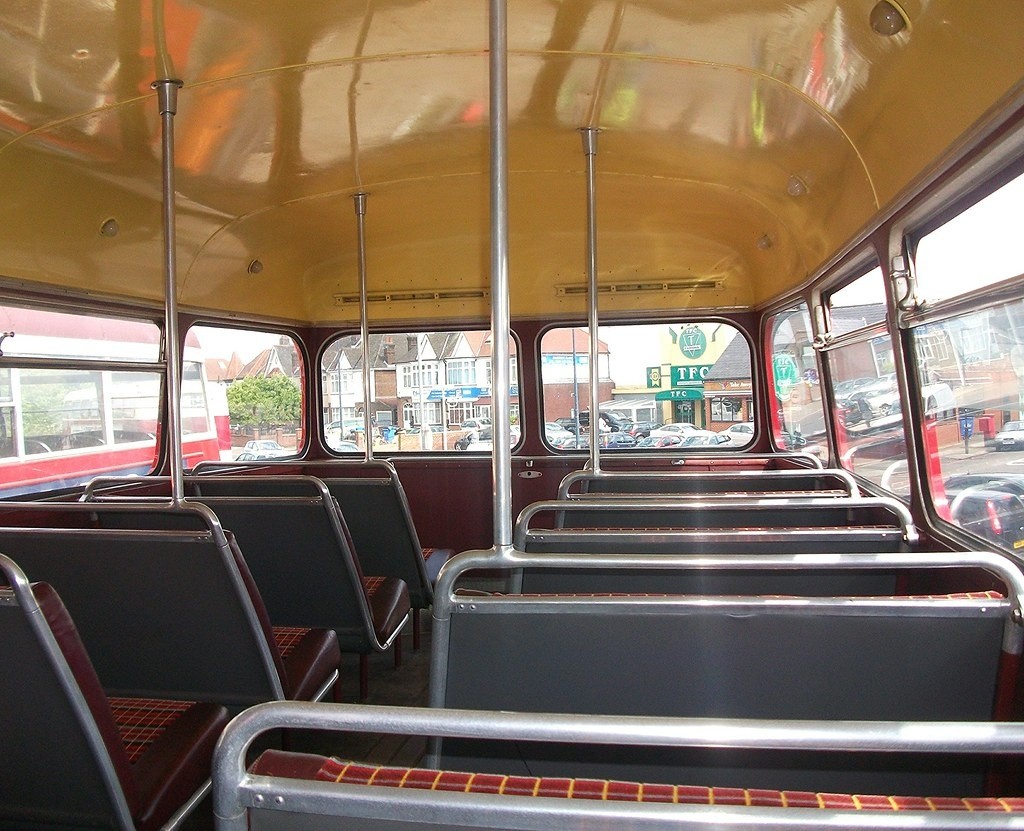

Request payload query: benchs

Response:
[0,459,457,831]
[211,452,1024,831]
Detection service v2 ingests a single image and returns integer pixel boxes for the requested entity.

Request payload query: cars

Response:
[994,420,1024,451]
[234,418,451,462]
[829,372,960,423]
[453,411,754,449]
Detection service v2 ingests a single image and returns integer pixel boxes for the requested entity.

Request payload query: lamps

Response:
[248,258,263,274]
[867,0,912,36]
[98,217,119,237]
[754,234,774,250]
[787,175,810,196]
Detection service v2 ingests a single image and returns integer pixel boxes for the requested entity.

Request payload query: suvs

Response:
[903,471,1024,561]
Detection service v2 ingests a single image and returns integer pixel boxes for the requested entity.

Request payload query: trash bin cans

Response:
[382,428,397,443]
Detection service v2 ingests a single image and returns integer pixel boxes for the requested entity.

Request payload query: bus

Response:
[0,300,224,495]
